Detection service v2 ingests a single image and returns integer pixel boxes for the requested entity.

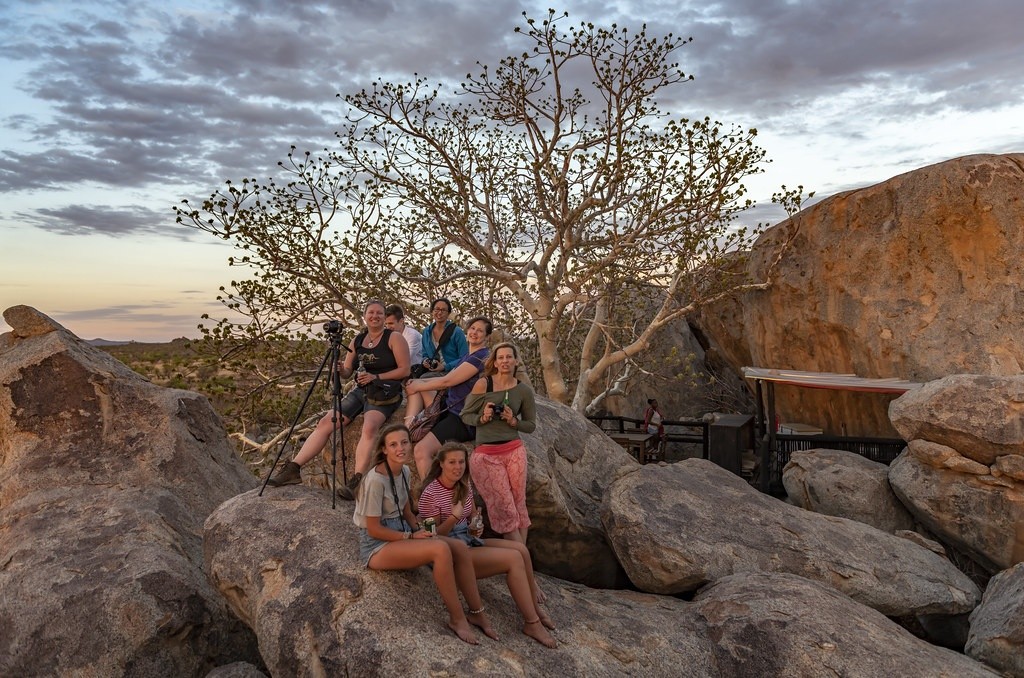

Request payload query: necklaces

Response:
[366,330,384,348]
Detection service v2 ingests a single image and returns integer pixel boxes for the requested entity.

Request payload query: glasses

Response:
[433,308,448,314]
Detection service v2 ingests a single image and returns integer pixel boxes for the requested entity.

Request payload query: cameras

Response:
[489,404,504,415]
[323,320,342,334]
[426,358,438,369]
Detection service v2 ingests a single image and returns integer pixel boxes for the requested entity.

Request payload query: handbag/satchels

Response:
[407,396,441,442]
[365,378,401,405]
[410,363,427,378]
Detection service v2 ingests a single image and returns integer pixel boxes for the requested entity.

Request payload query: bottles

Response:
[470,507,482,536]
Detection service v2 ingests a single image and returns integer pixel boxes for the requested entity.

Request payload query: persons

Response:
[458,342,547,602]
[415,441,559,648]
[383,304,425,406]
[644,398,665,458]
[403,316,493,491]
[352,423,501,645]
[403,298,471,429]
[266,300,412,502]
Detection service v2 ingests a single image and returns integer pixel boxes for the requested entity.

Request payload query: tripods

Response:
[257,335,353,510]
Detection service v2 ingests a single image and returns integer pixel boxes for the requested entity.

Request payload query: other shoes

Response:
[261,459,302,486]
[337,471,363,499]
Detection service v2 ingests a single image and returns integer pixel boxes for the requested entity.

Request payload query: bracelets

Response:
[451,514,460,521]
[409,534,413,540]
